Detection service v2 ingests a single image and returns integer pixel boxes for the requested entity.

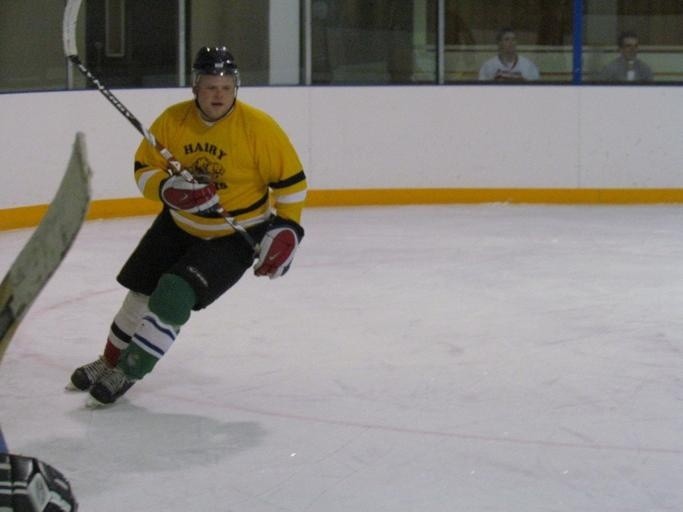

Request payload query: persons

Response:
[476,27,539,80]
[599,30,653,81]
[75,43,309,404]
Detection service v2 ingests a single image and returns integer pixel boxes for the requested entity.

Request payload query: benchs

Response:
[414,45,682,84]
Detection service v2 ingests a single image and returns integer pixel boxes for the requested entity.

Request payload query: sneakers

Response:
[72,357,110,392]
[89,368,134,405]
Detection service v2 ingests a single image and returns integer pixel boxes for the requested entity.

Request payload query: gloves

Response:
[158,172,219,216]
[253,216,304,280]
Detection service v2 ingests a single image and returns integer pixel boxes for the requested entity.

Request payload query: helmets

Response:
[192,46,241,87]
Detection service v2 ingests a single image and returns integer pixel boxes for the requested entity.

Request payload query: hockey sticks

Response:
[60,1,263,258]
[0,132,92,367]
